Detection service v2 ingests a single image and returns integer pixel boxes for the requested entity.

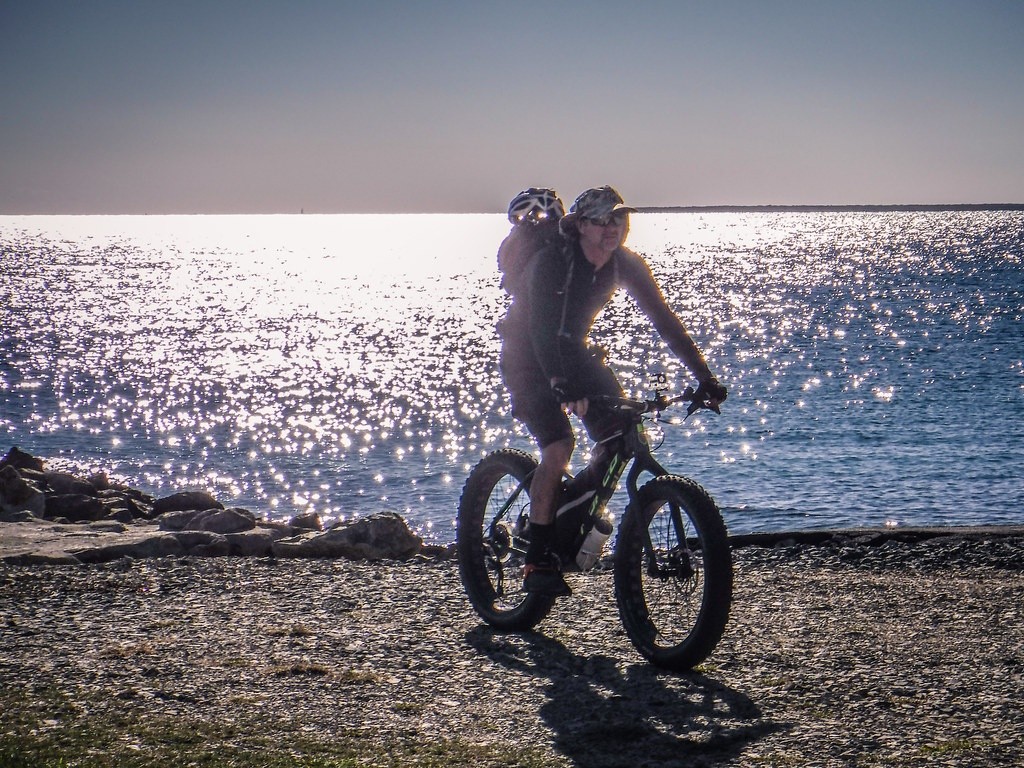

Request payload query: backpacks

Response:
[497,224,565,296]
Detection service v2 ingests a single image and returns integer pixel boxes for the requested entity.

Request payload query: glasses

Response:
[580,213,626,225]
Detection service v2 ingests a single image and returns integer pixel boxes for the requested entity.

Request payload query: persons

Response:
[499,186,727,595]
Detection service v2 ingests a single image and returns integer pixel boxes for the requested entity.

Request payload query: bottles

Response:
[576,519,613,570]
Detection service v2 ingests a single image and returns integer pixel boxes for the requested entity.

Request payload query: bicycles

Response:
[455,374,734,673]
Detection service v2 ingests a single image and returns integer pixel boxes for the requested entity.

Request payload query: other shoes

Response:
[562,476,613,534]
[523,564,572,596]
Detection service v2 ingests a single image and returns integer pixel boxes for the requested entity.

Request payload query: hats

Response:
[559,185,638,232]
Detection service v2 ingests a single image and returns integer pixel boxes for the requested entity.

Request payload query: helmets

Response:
[506,186,566,227]
